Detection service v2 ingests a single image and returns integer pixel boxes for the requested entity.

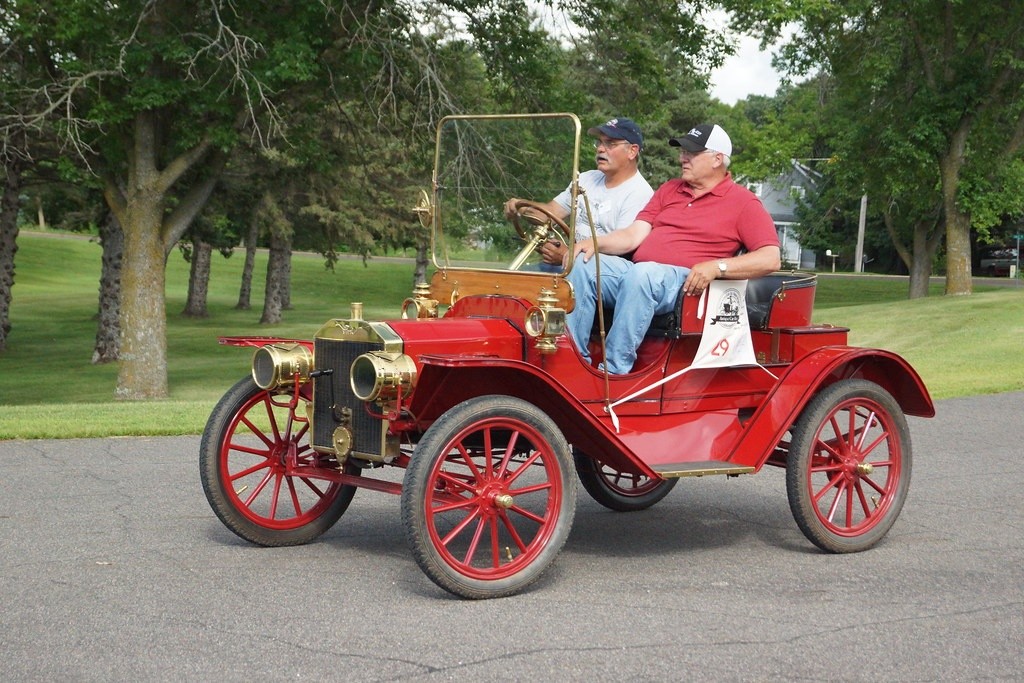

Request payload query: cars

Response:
[202,113,936,601]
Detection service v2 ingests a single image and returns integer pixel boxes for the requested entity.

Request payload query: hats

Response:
[586,119,643,150]
[669,124,732,157]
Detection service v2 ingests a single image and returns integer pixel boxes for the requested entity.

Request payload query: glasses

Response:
[591,138,629,149]
[678,149,713,158]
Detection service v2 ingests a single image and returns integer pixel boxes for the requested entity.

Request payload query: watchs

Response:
[718,259,727,277]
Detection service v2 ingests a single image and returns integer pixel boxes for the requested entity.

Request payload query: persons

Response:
[504,117,655,274]
[561,125,780,376]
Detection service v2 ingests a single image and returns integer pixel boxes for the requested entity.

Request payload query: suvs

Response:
[980,249,1024,278]
